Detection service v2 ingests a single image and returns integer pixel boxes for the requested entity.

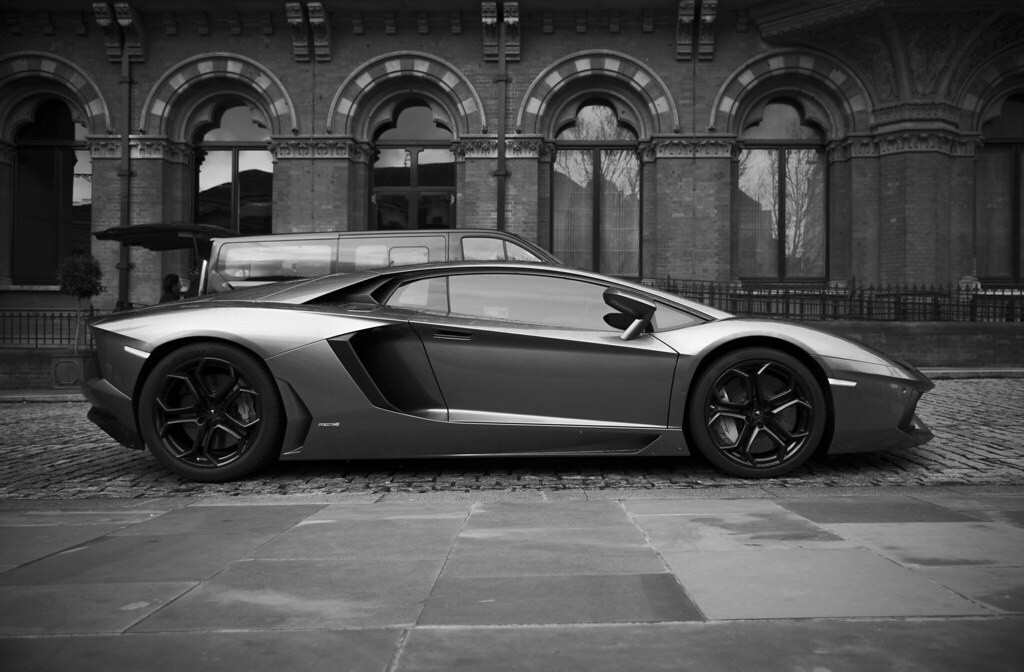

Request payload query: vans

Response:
[202,230,570,296]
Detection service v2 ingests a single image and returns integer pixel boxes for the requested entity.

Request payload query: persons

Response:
[158,273,183,303]
[184,265,201,298]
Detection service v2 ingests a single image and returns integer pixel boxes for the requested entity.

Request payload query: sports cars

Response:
[78,264,936,484]
